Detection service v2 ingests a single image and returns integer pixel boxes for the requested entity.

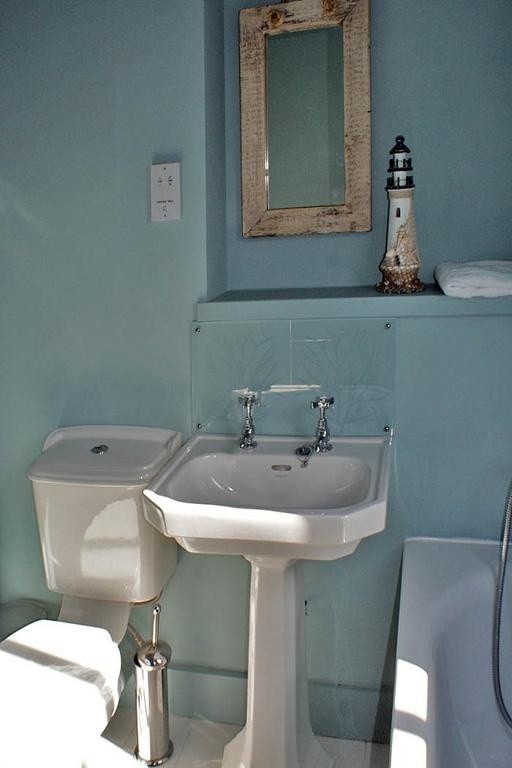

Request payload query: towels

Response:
[435,259,512,299]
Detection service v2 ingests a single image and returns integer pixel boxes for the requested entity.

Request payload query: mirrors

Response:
[240,1,373,238]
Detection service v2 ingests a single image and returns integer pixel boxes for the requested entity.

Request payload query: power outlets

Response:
[149,160,181,221]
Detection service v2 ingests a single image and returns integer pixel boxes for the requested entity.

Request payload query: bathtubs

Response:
[387,536,512,768]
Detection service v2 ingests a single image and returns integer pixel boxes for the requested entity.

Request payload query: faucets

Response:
[238,394,258,449]
[310,395,335,454]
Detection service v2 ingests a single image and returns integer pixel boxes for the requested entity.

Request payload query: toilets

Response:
[0,425,180,767]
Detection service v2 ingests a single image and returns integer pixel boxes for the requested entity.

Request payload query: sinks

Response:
[143,430,393,563]
